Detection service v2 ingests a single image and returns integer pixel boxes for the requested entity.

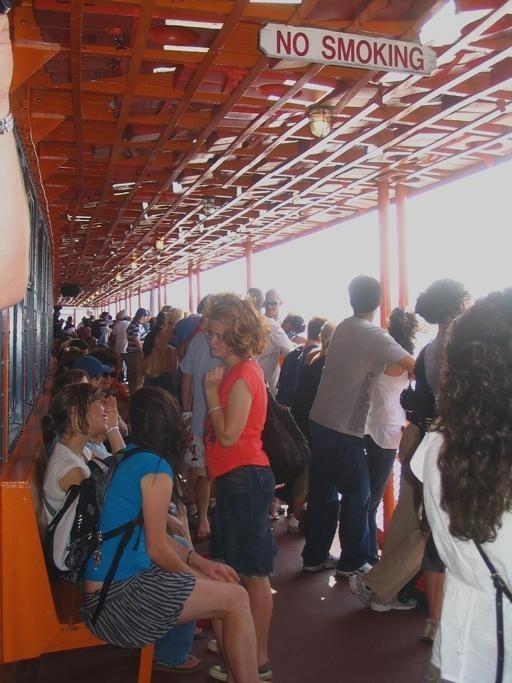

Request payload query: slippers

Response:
[151,652,203,678]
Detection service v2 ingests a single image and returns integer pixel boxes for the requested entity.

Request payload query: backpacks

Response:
[43,446,172,622]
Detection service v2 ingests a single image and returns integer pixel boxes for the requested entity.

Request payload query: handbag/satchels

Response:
[108,334,117,350]
[262,386,309,484]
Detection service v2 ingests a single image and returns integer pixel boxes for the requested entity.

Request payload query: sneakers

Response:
[184,495,220,544]
[303,553,420,613]
[271,502,306,533]
[420,618,438,641]
[206,636,218,651]
[420,662,440,683]
[210,660,274,683]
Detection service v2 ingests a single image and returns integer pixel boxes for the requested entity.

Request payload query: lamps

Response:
[302,103,335,139]
[202,195,216,215]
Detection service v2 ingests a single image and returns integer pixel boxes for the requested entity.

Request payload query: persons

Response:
[411,277,467,644]
[78,382,261,682]
[46,277,417,674]
[196,292,276,680]
[408,283,512,682]
[347,343,436,612]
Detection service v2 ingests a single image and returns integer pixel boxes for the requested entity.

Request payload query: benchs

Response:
[0,373,157,682]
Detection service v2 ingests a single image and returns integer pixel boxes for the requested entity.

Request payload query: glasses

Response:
[261,300,280,308]
